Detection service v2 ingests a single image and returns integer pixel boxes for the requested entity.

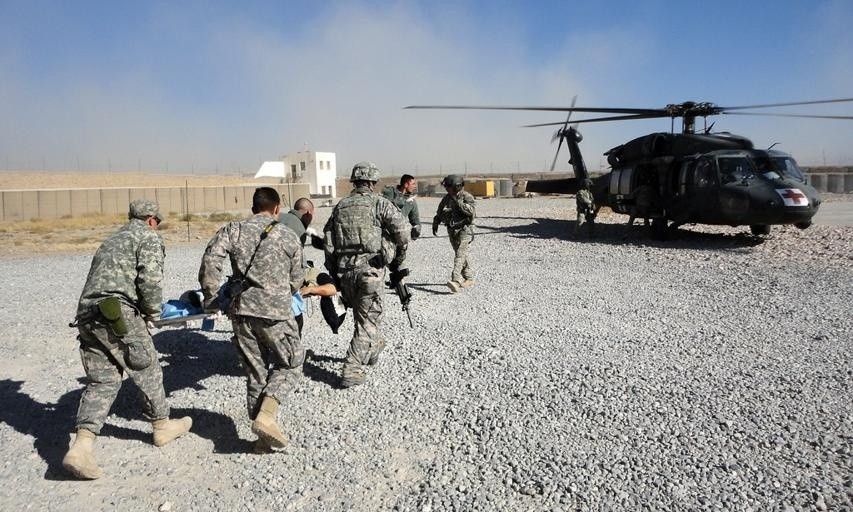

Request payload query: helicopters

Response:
[400,94,853,243]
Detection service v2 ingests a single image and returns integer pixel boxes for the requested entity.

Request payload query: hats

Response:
[127,197,167,223]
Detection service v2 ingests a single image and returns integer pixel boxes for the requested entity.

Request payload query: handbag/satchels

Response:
[220,274,245,319]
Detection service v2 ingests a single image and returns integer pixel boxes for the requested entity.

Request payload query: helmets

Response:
[347,159,384,185]
[439,173,466,192]
[580,178,595,189]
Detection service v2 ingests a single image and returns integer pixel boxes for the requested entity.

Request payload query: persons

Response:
[60,200,193,481]
[323,161,412,389]
[198,187,306,455]
[625,179,650,230]
[278,197,313,339]
[379,174,420,291]
[431,174,475,292]
[576,174,595,227]
[160,264,337,316]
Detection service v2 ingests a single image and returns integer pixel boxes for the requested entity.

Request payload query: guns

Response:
[388,264,414,329]
[91,298,128,337]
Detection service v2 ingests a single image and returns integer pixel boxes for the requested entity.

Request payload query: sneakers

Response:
[367,337,386,365]
[339,370,367,389]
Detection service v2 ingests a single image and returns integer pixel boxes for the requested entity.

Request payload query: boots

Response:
[251,393,290,453]
[461,277,477,288]
[149,415,193,447]
[446,279,461,293]
[61,428,105,481]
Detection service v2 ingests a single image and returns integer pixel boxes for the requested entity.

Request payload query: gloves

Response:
[394,248,409,266]
